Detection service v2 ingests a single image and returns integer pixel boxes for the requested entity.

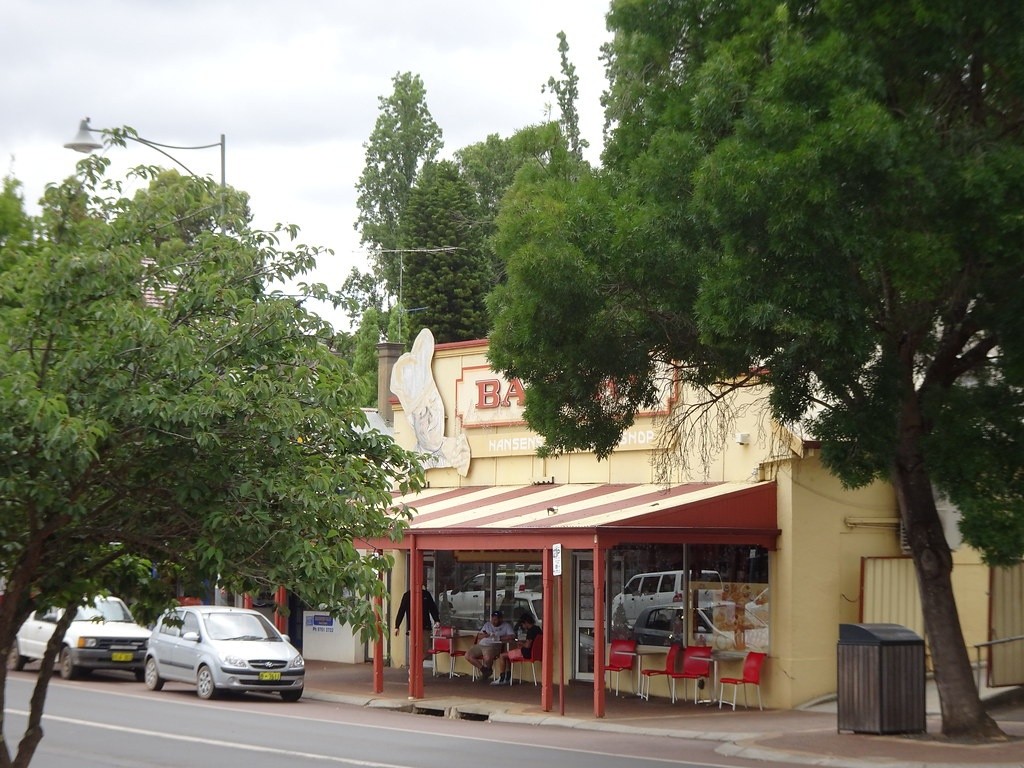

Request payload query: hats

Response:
[492,611,504,616]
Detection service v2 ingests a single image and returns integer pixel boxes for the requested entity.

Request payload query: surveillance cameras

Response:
[736,433,750,446]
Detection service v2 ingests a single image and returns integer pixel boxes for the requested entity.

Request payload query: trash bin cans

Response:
[837,623,927,734]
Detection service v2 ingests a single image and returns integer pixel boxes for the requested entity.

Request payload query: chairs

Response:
[451,633,482,682]
[604,639,637,697]
[473,654,501,682]
[514,607,527,618]
[427,625,456,679]
[653,613,669,630]
[510,635,543,686]
[672,646,714,705]
[640,643,682,702]
[718,650,768,712]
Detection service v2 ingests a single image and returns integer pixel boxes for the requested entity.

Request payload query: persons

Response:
[463,610,544,687]
[393,567,440,691]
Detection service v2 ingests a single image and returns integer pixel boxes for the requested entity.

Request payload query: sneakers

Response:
[490,678,510,685]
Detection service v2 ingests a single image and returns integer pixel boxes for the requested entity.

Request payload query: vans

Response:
[612,568,721,629]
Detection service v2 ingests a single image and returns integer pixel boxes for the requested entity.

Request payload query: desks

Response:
[488,639,526,683]
[689,654,744,708]
[614,649,667,696]
[430,633,473,679]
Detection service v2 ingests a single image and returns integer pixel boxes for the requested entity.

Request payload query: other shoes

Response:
[480,667,492,679]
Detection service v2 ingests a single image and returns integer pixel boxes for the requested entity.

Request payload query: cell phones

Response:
[514,638,519,642]
[482,630,489,638]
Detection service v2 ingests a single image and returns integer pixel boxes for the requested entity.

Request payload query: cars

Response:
[495,593,543,642]
[145,604,304,705]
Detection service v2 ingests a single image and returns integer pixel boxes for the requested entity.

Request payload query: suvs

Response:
[6,595,149,682]
[439,571,542,623]
